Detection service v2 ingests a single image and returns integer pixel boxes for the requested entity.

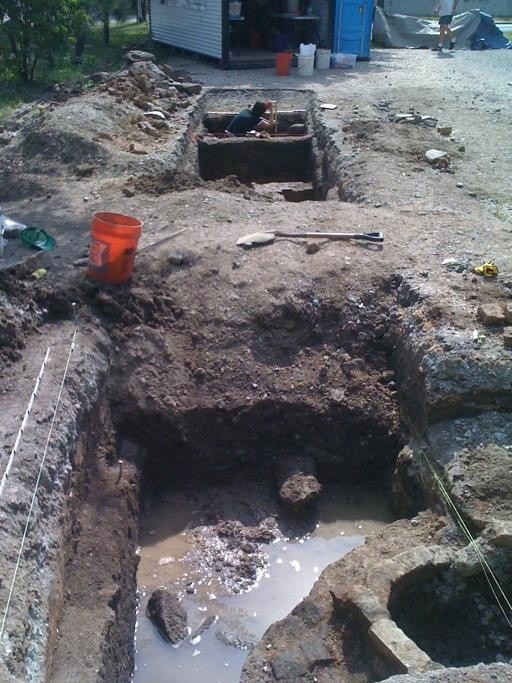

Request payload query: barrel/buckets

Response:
[316,50,331,69]
[297,54,315,76]
[276,51,292,76]
[87,211,143,284]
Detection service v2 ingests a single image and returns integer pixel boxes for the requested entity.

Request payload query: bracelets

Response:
[435,9,438,11]
[453,7,456,9]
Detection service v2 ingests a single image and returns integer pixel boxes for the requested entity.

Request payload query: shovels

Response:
[236,227,383,247]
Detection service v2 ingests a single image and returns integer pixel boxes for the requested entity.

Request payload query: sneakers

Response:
[432,46,443,51]
[449,39,457,50]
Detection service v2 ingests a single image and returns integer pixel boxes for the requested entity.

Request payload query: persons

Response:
[431,0,459,50]
[224,101,273,137]
